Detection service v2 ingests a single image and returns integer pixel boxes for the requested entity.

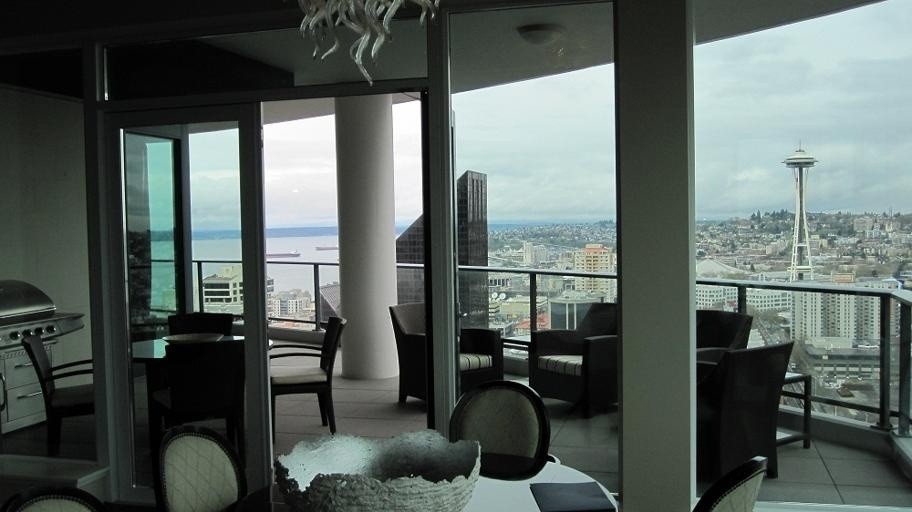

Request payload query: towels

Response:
[530,482,616,512]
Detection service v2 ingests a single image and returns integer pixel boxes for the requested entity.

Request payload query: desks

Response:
[221,454,621,512]
[132,335,274,417]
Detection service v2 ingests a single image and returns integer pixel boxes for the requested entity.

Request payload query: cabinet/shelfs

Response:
[0,338,58,435]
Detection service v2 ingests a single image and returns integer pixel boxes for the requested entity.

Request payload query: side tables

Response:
[768,372,812,479]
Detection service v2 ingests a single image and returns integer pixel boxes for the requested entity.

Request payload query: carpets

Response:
[693,496,912,512]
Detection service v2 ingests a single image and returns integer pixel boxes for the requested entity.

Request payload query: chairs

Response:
[450,381,561,464]
[21,335,96,459]
[528,303,618,418]
[148,340,245,470]
[693,311,794,512]
[0,483,111,512]
[167,312,234,336]
[268,317,347,443]
[389,302,503,402]
[154,425,248,512]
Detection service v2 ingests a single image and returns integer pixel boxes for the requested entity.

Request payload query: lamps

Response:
[295,0,441,88]
[517,24,567,46]
